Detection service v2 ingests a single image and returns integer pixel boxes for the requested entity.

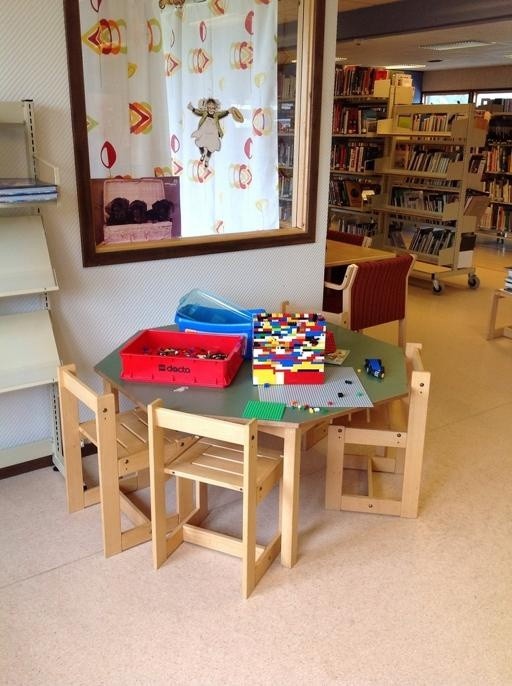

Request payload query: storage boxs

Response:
[175,289,267,361]
[102,179,172,243]
[120,330,244,388]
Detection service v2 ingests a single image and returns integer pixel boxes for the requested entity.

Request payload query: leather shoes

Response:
[104,198,171,224]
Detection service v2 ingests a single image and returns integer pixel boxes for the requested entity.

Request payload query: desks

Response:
[95,322,409,569]
[486,289,512,341]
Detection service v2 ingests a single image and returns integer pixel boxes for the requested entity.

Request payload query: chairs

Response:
[324,342,431,518]
[325,254,416,347]
[147,399,281,598]
[57,363,194,558]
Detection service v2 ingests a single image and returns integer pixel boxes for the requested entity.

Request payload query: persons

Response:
[188,98,235,166]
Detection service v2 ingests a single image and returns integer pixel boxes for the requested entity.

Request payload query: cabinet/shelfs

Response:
[0,99,65,481]
[278,86,512,296]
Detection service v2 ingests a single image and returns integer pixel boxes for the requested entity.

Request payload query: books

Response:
[279,63,511,257]
[0,177,59,196]
[1,192,58,202]
[502,266,512,294]
